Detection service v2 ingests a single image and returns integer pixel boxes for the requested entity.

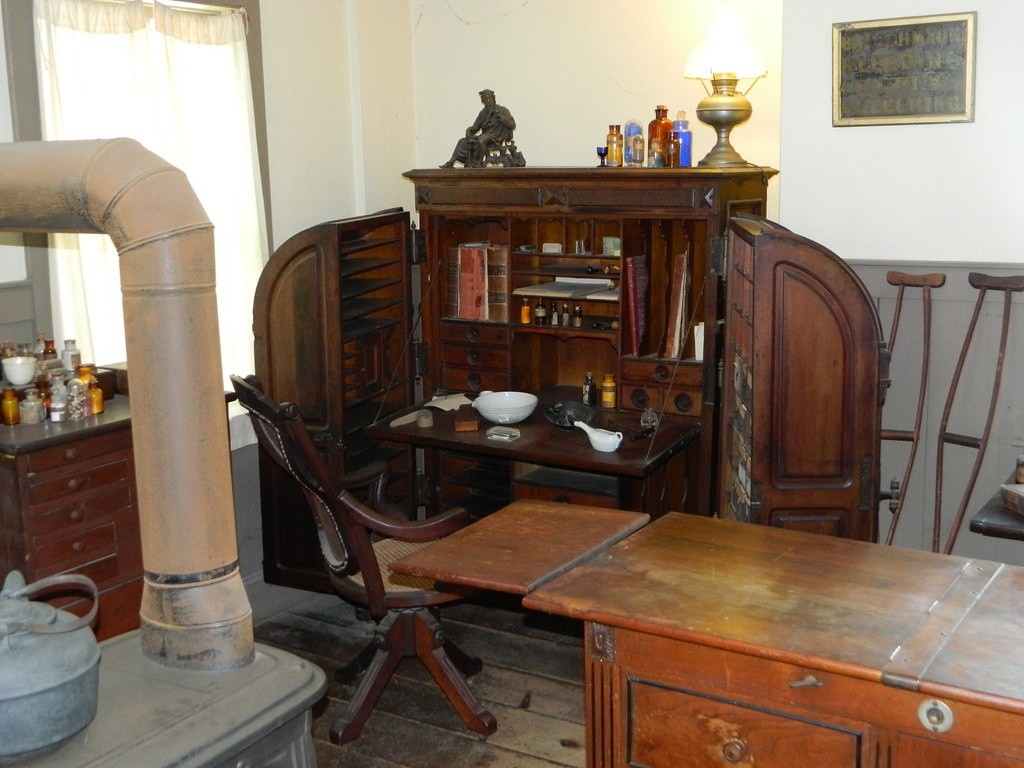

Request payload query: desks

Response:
[369,391,702,522]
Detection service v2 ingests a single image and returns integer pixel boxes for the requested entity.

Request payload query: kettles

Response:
[0,569,102,764]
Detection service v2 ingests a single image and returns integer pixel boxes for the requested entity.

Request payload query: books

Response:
[627,255,650,357]
[445,241,508,323]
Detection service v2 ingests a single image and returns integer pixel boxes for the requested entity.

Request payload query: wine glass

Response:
[596,146,608,167]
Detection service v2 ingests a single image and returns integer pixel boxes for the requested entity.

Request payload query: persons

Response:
[438,89,516,169]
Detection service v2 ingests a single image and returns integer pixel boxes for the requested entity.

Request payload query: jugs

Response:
[575,420,623,452]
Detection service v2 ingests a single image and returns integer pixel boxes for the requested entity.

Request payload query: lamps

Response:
[683,26,772,169]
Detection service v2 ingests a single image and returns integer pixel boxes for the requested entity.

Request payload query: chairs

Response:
[229,370,499,743]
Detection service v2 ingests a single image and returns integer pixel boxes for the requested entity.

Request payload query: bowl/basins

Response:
[2,357,38,386]
[471,390,538,424]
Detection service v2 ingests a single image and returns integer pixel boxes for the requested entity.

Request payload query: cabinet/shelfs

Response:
[255,166,889,636]
[0,388,145,643]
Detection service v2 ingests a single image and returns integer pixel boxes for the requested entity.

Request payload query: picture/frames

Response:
[831,10,975,124]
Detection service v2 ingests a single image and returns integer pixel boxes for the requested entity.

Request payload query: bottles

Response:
[647,105,673,167]
[550,302,559,326]
[602,374,617,409]
[665,131,680,168]
[0,334,105,425]
[534,296,547,326]
[573,304,582,327]
[520,298,531,325]
[562,304,570,327]
[582,372,597,407]
[673,110,692,168]
[606,124,623,168]
